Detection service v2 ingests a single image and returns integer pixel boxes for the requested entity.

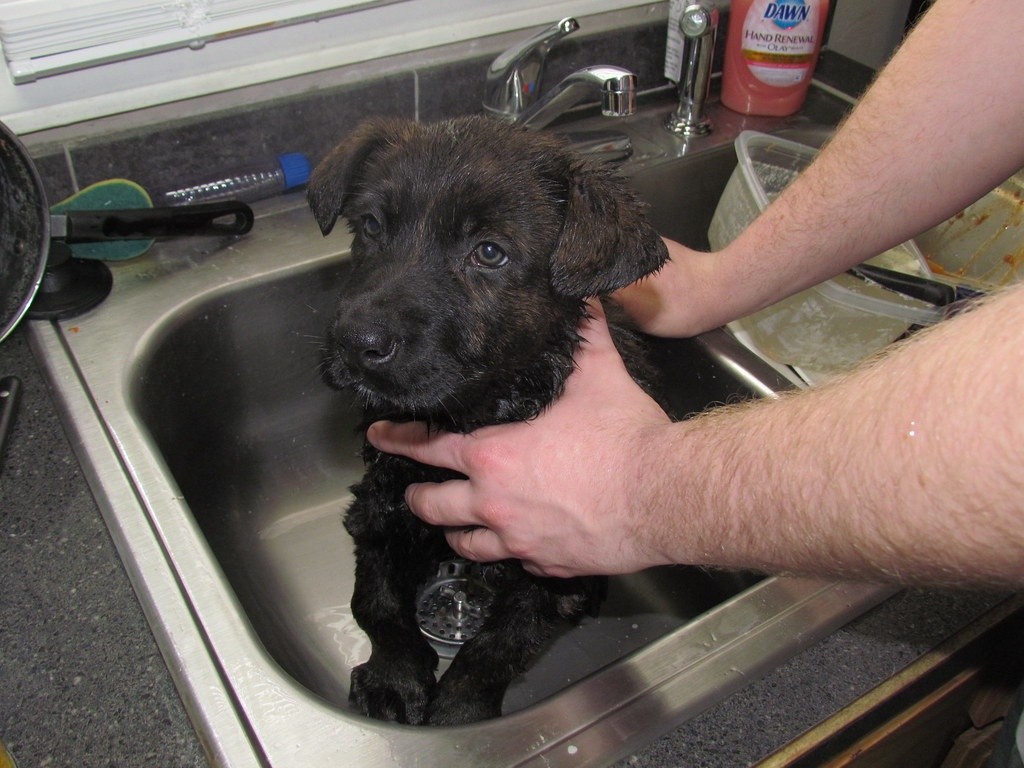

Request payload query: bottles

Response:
[720,0,830,117]
[153,151,313,207]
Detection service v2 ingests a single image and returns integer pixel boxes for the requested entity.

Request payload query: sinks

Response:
[619,132,1023,373]
[119,254,782,747]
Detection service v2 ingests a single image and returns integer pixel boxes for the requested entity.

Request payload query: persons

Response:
[369,1,1024,589]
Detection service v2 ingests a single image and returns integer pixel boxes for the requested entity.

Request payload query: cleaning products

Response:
[719,0,830,117]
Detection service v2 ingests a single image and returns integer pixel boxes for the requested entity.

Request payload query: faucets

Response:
[479,14,639,164]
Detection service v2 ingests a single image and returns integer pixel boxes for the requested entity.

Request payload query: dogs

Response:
[303,116,726,727]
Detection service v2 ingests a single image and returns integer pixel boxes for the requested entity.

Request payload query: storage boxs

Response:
[707,128,948,378]
